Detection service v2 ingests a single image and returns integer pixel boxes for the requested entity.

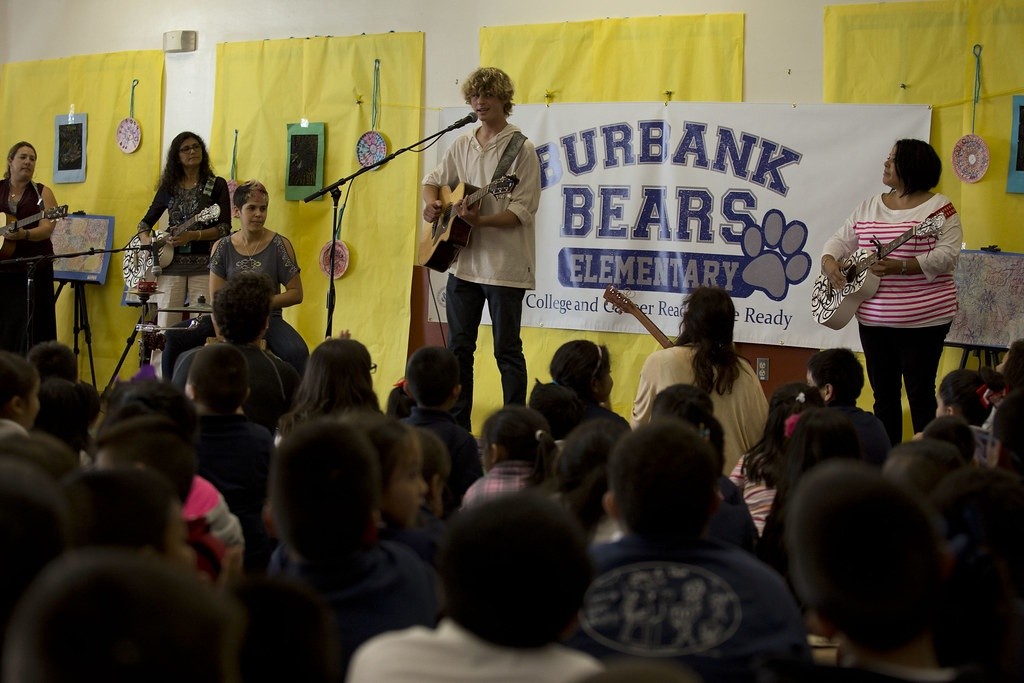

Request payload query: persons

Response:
[0,273,1024,683]
[822,138,962,445]
[422,67,541,432]
[137,132,232,378]
[161,181,309,384]
[0,142,58,356]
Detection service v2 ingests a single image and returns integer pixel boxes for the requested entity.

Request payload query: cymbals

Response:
[125,290,166,295]
[153,303,214,313]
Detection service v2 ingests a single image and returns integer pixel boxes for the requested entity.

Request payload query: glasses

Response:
[178,143,201,154]
[368,364,378,374]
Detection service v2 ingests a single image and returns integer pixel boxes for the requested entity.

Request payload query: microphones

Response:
[447,112,478,131]
[151,230,162,277]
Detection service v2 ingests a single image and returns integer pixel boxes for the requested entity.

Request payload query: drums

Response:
[206,335,267,350]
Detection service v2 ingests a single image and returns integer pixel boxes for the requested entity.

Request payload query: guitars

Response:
[0,204,69,259]
[416,175,517,274]
[810,212,947,330]
[602,283,674,350]
[122,202,221,290]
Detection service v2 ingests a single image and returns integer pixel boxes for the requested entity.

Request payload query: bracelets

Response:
[197,230,201,240]
[822,258,830,266]
[25,231,30,240]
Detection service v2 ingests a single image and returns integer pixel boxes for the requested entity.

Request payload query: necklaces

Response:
[242,229,264,261]
